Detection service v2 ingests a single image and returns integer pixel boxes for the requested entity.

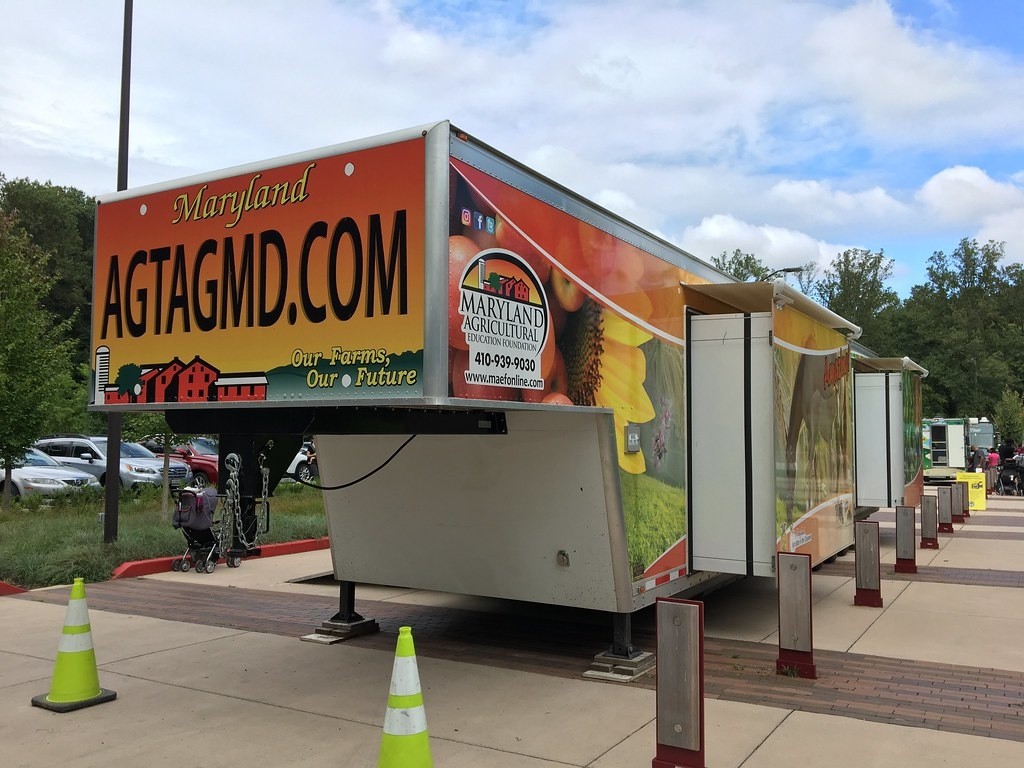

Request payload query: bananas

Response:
[596,252,656,473]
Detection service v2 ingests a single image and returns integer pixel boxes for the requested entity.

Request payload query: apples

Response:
[449,164,584,407]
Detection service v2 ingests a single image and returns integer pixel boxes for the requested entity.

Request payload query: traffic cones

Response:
[31,577,117,713]
[374,626,436,768]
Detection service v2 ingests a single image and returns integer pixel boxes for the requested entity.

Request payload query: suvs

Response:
[32,433,194,493]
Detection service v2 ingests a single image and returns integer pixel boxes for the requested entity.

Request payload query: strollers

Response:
[992,461,1024,497]
[169,488,242,573]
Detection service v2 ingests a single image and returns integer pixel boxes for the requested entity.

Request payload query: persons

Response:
[968,439,1024,496]
[306,438,318,482]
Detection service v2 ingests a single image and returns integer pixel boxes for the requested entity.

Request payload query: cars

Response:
[135,434,319,489]
[0,446,103,508]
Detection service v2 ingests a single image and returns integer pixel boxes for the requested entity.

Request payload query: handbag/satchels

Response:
[975,467,983,473]
